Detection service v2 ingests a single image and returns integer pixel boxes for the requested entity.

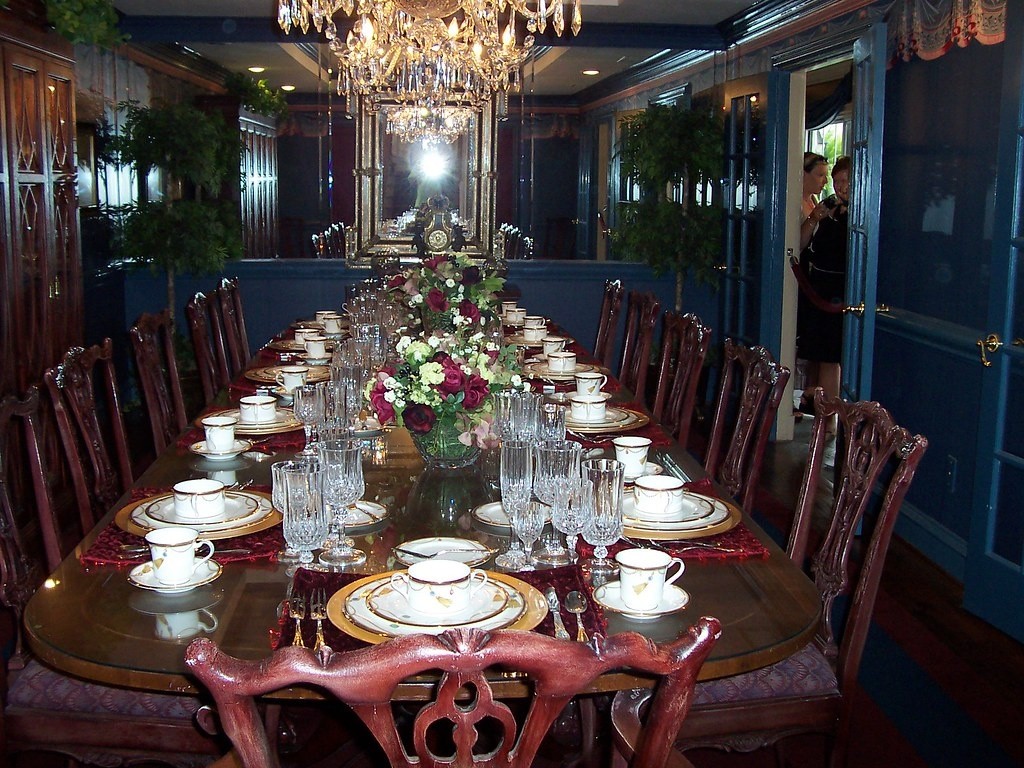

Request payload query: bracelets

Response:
[807,217,816,226]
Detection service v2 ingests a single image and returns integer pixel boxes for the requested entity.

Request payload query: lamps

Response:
[382,106,481,152]
[277,0,583,111]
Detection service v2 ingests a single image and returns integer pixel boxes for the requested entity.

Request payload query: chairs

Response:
[0,272,928,768]
[311,221,535,259]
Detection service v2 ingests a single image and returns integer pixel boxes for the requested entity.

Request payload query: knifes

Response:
[546,587,571,641]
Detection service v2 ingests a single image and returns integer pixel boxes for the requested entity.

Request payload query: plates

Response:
[498,313,742,540]
[126,556,223,594]
[471,501,552,527]
[592,580,691,620]
[326,536,549,644]
[115,320,352,540]
[130,588,222,614]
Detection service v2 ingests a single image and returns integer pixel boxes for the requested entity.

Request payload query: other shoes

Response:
[799,393,815,415]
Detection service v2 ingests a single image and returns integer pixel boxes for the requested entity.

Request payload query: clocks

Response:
[422,193,455,252]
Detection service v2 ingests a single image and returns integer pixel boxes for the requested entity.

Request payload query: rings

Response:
[820,214,821,215]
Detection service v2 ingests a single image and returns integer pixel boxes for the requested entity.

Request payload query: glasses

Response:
[836,155,850,164]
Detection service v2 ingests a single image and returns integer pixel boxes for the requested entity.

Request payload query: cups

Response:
[391,559,487,614]
[172,311,343,518]
[615,549,686,610]
[154,608,218,641]
[145,527,214,585]
[501,301,684,517]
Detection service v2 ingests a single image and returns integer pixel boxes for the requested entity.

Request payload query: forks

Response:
[225,479,253,490]
[620,534,736,553]
[567,429,623,443]
[390,546,500,559]
[250,434,273,445]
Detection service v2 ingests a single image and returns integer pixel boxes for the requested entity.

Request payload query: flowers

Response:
[377,252,500,330]
[360,334,520,434]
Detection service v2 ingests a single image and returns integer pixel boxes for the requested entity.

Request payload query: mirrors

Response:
[74,14,726,267]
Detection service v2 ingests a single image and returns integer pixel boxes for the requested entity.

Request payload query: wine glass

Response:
[271,278,403,570]
[495,388,625,573]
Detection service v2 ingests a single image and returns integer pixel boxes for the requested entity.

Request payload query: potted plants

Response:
[611,102,726,435]
[94,95,247,438]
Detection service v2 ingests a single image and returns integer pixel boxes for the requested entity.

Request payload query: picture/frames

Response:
[354,83,497,259]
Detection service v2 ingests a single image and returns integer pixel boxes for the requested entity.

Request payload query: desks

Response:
[23,313,822,768]
[380,208,473,241]
[366,244,483,255]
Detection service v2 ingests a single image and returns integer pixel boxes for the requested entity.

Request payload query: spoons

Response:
[565,591,589,642]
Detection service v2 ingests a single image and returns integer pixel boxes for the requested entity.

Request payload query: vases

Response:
[407,416,493,469]
[420,309,477,339]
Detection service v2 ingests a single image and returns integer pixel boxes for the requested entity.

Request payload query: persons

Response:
[794,152,851,446]
